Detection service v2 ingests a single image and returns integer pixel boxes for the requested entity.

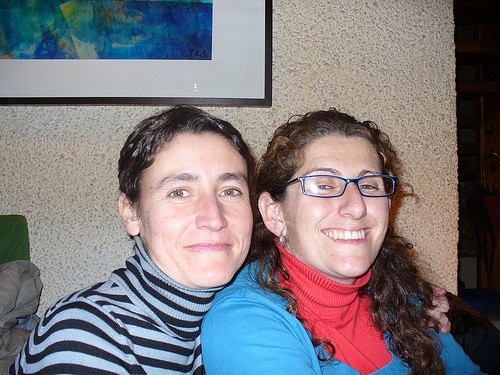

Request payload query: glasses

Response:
[275,173,399,198]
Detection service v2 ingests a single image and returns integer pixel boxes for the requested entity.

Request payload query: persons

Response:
[200,106,487,374]
[0,103,259,375]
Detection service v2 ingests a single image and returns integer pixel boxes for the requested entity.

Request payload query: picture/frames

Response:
[1,0,273,108]
